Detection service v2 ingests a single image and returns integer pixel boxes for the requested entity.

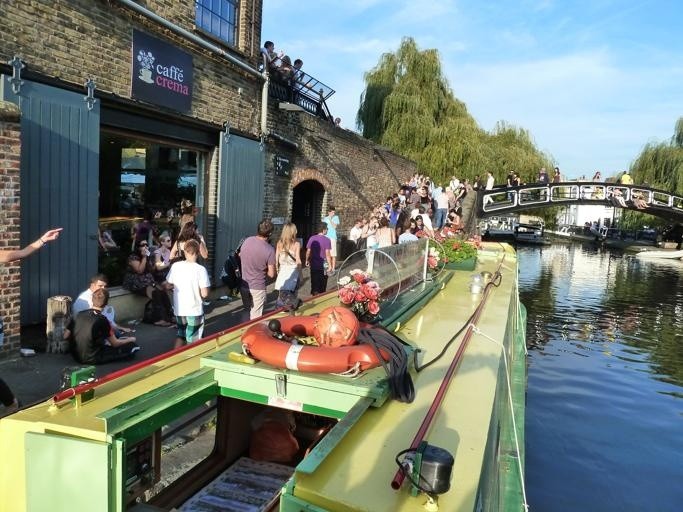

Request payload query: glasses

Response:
[140,244,148,247]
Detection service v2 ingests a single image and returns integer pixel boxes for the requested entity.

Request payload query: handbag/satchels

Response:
[144,300,155,324]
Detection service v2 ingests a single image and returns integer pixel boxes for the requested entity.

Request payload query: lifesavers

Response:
[242,316,394,373]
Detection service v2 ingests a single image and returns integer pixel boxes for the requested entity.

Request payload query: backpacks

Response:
[222,242,242,290]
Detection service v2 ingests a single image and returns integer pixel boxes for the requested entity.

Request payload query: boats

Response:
[482,230,518,250]
[0,239,531,512]
[93,216,181,265]
[513,220,548,245]
[635,251,682,261]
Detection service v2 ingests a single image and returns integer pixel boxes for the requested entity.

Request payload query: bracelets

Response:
[38,237,46,245]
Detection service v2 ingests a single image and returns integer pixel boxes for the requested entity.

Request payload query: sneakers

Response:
[154,320,171,327]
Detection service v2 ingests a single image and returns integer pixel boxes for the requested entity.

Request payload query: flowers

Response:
[333,269,387,321]
[411,225,486,275]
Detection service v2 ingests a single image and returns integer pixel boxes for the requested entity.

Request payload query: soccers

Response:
[313,306,359,349]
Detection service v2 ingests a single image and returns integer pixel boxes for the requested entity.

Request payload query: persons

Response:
[305,222,332,296]
[260,40,342,126]
[447,171,649,228]
[233,219,275,323]
[0,226,63,416]
[322,206,339,273]
[70,272,135,347]
[63,290,140,364]
[128,199,211,349]
[275,223,302,310]
[346,172,456,262]
